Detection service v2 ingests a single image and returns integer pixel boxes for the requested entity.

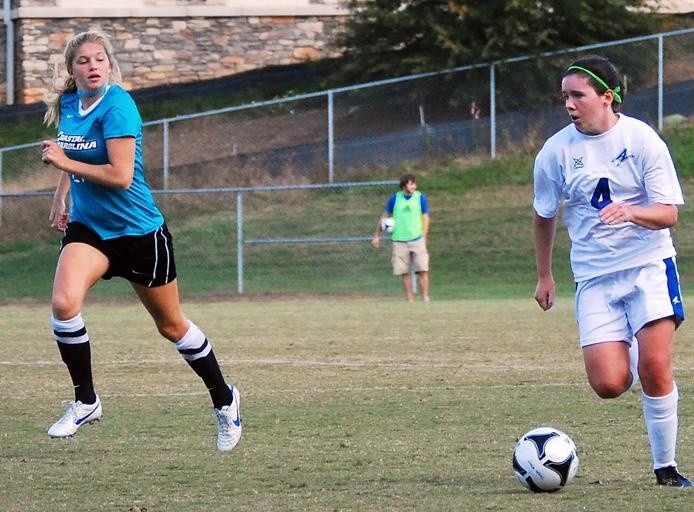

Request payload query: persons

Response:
[529,56,694,488]
[38,30,242,451]
[372,174,430,301]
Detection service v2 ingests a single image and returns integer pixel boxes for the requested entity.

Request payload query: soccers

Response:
[511,426,578,493]
[381,216,394,233]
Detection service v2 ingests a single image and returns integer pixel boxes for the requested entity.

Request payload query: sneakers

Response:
[213,383,242,452]
[653,465,693,487]
[47,388,103,440]
